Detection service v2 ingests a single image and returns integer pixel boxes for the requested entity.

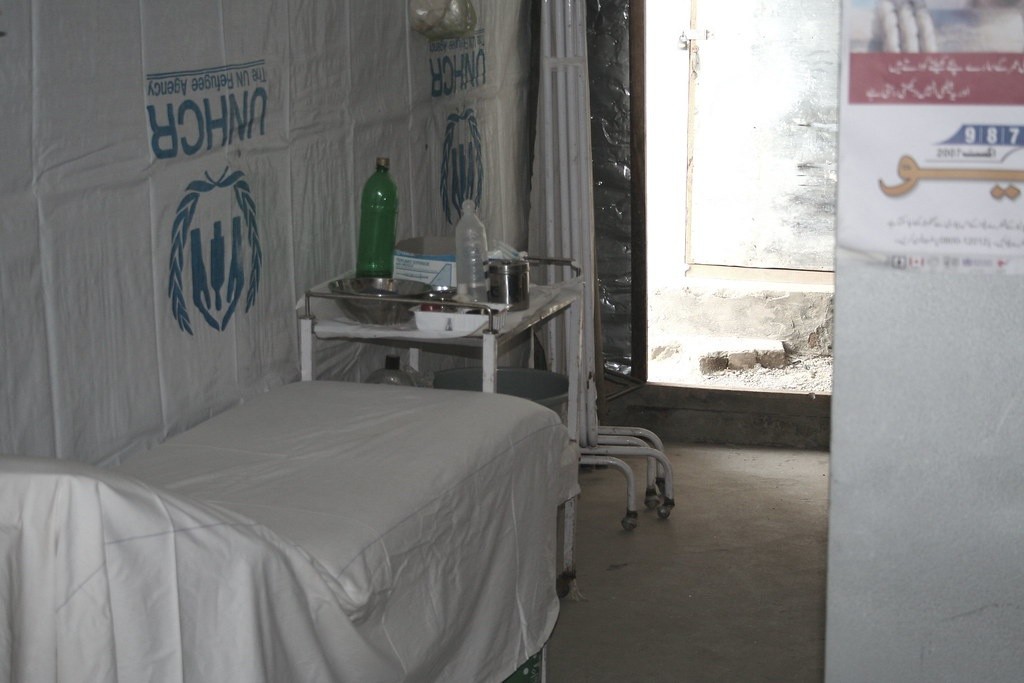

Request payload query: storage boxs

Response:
[392,235,503,291]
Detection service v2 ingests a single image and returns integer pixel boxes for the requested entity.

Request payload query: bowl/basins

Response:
[409,303,514,337]
[329,278,433,326]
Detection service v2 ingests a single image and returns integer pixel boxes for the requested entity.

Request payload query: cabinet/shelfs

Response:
[300,257,583,595]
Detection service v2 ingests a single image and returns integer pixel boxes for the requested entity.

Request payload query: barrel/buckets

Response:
[483,257,530,311]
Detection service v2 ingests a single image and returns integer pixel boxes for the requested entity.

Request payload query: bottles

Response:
[456,199,488,304]
[355,157,397,277]
[367,354,415,386]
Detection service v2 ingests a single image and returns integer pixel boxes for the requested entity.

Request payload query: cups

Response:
[423,285,457,313]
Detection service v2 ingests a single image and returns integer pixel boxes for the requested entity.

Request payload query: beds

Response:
[0,380,580,683]
